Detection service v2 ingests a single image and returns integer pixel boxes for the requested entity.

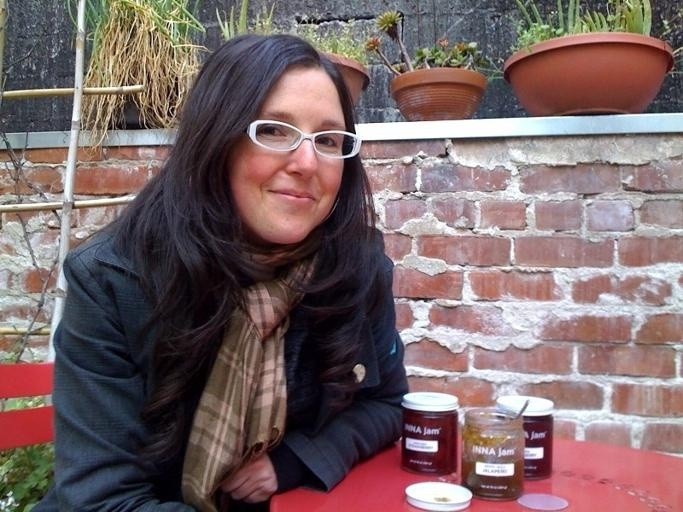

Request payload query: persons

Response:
[30,31,414,512]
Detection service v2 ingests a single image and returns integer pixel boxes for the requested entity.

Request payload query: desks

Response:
[270,428,682,512]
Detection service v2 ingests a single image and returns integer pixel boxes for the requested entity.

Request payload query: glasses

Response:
[243,119,362,159]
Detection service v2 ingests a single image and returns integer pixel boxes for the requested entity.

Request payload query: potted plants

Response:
[298,0,674,122]
